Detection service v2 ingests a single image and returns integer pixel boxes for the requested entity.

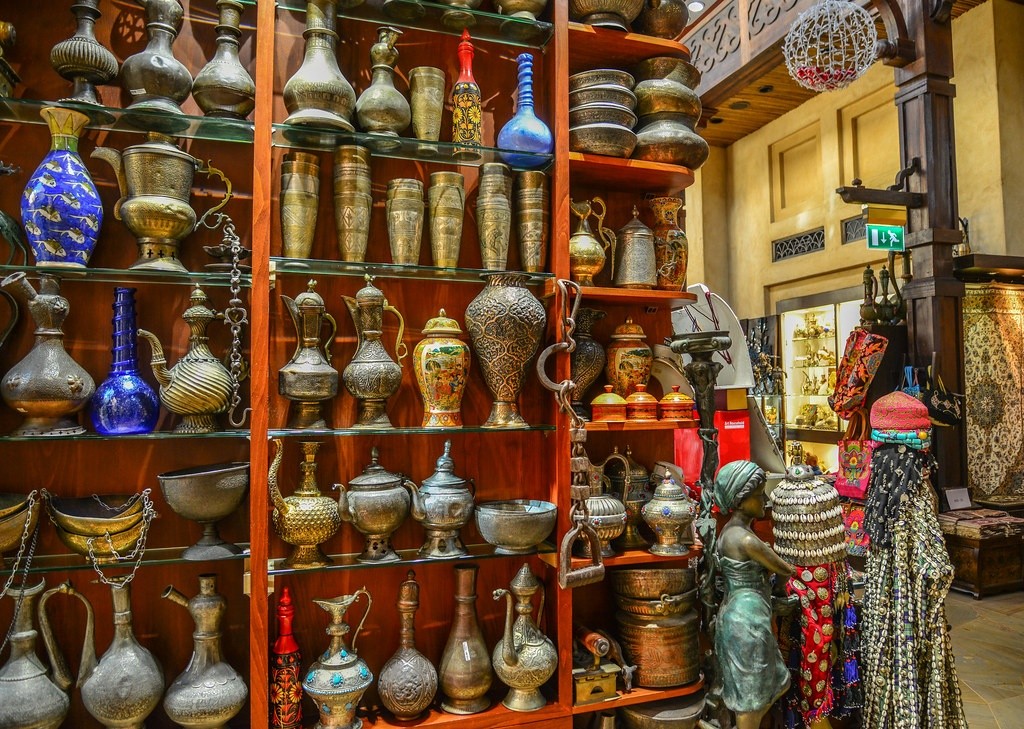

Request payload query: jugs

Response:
[340,272,409,429]
[161,573,248,729]
[278,279,340,430]
[301,586,374,729]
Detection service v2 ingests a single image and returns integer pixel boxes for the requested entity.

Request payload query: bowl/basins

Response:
[474,499,558,549]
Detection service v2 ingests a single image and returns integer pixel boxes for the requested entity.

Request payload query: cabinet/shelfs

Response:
[264,0,559,728]
[755,392,784,432]
[563,21,707,718]
[789,334,837,430]
[0,0,250,729]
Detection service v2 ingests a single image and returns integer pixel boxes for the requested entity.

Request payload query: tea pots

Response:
[136,282,251,434]
[267,438,475,570]
[90,132,234,272]
[492,562,558,712]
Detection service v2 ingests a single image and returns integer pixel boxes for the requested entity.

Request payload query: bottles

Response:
[91,286,161,436]
[608,316,654,400]
[648,197,688,291]
[497,53,554,154]
[452,27,481,144]
[569,307,607,422]
[51,0,411,137]
[438,562,494,714]
[464,272,547,427]
[641,467,695,557]
[270,586,304,729]
[21,107,103,268]
[377,570,438,720]
[413,307,471,429]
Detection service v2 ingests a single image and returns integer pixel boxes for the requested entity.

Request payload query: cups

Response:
[477,162,512,269]
[385,172,465,268]
[279,152,320,258]
[408,66,445,140]
[333,145,373,262]
[516,170,549,272]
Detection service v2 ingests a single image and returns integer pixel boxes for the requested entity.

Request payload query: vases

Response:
[88,286,161,435]
[495,52,553,169]
[649,196,689,290]
[20,104,106,277]
[436,562,495,715]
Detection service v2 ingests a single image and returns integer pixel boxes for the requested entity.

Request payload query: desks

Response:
[942,533,1024,600]
